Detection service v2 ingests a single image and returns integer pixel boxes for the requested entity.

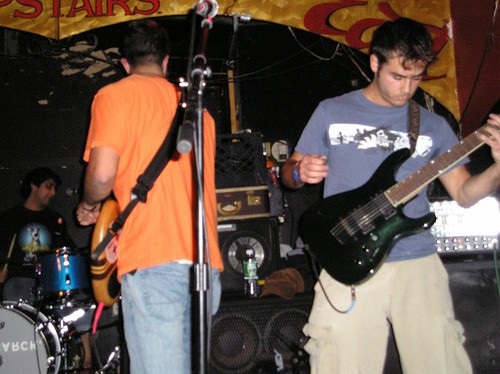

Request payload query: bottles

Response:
[243,249,259,298]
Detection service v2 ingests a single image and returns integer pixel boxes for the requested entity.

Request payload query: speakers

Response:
[191,219,317,374]
[444,258,500,374]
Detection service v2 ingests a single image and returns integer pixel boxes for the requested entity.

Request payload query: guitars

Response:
[91,200,122,307]
[297,122,494,285]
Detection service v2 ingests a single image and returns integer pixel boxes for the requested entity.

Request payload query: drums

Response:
[0,299,66,374]
[34,246,94,307]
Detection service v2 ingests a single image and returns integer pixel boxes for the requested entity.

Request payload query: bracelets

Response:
[82,205,97,212]
[292,161,304,186]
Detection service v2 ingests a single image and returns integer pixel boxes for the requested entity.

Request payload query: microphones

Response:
[195,0,219,18]
[53,218,62,243]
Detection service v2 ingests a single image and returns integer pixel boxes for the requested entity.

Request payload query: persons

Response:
[279,17,500,374]
[0,166,78,305]
[76,19,224,374]
